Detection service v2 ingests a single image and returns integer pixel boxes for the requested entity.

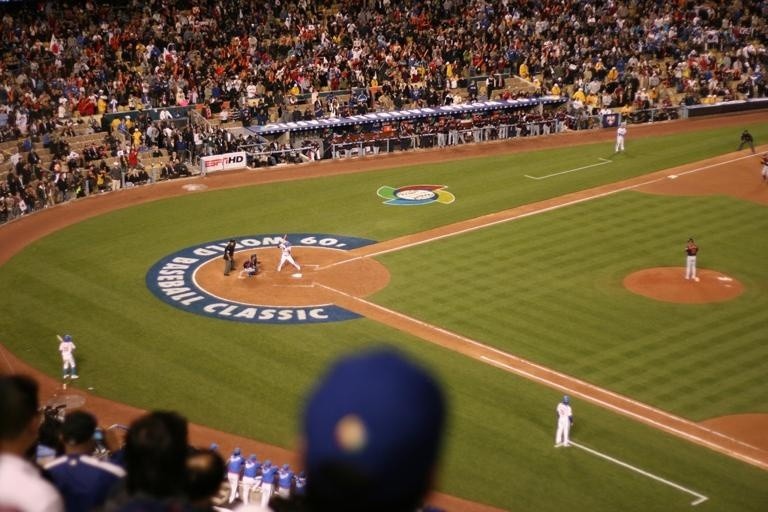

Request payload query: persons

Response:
[59,333,80,379]
[1,1,768,221]
[223,446,245,504]
[294,470,307,495]
[683,238,700,280]
[240,253,260,279]
[0,373,64,511]
[262,459,279,506]
[224,240,237,276]
[278,463,295,499]
[102,410,213,511]
[268,348,446,512]
[243,454,263,504]
[553,395,575,447]
[35,408,127,511]
[185,448,227,502]
[277,241,301,272]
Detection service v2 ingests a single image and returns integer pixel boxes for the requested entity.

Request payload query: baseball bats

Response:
[280,234,287,244]
[56,335,64,342]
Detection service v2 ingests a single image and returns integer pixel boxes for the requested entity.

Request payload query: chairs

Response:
[0,74,536,214]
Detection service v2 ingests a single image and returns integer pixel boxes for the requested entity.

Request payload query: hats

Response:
[234,447,305,477]
[306,355,447,463]
[211,444,217,449]
